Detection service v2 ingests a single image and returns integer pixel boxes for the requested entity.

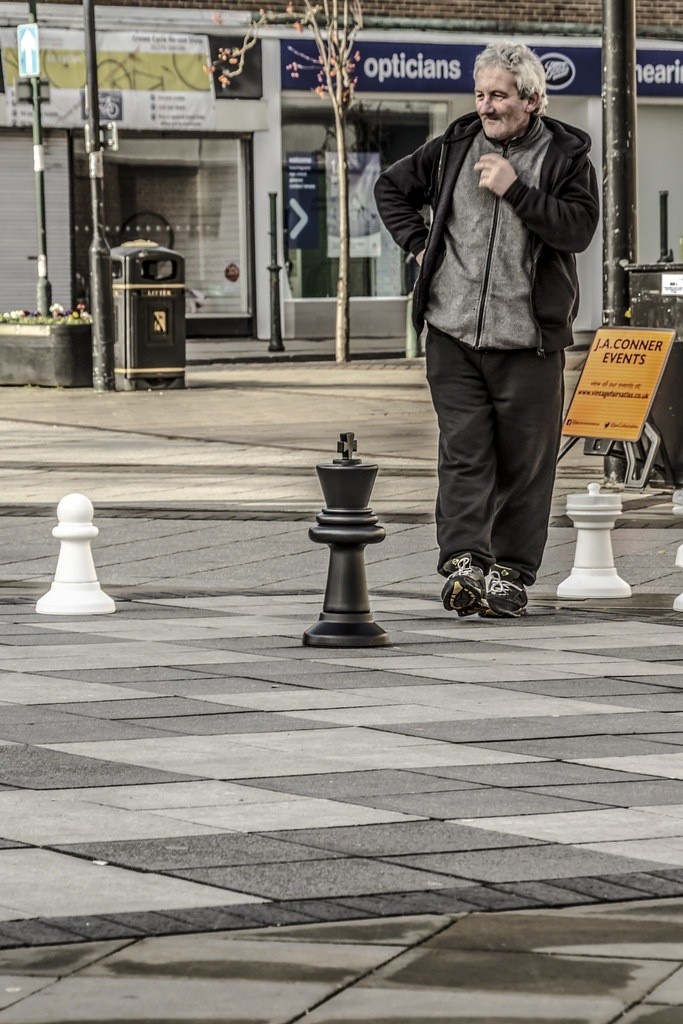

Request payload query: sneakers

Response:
[441,552,490,617]
[479,562,528,618]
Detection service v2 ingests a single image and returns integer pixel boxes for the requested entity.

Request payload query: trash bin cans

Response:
[625,259,683,476]
[110,239,187,392]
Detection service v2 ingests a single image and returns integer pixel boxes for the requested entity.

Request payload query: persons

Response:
[373,42,599,618]
